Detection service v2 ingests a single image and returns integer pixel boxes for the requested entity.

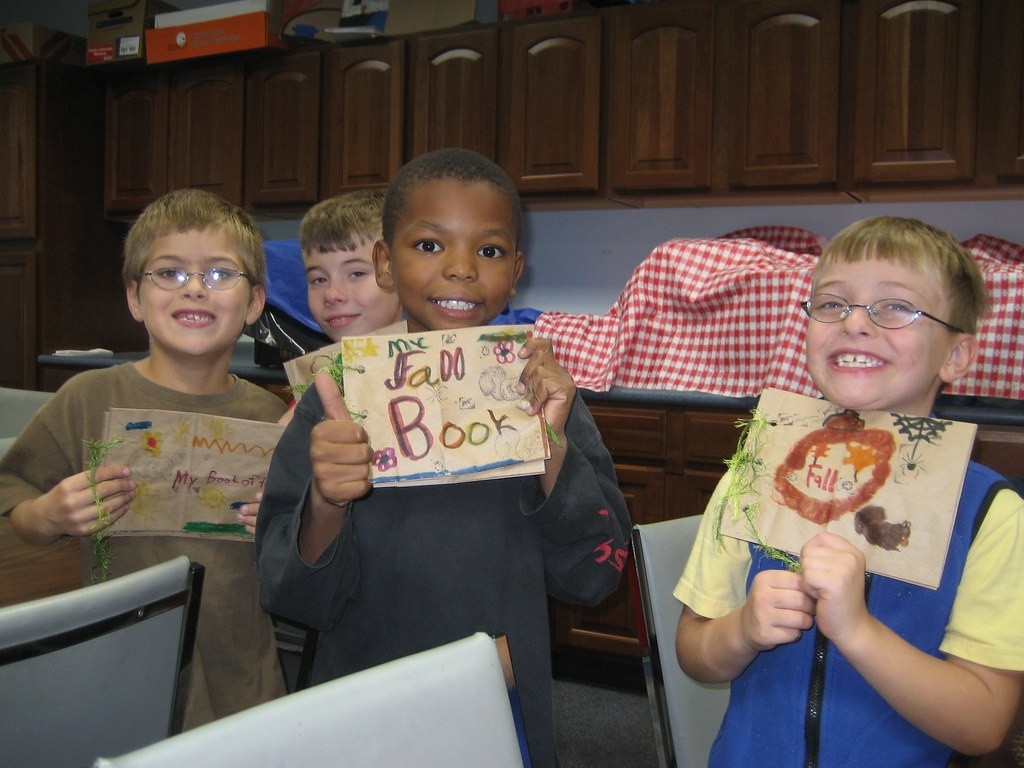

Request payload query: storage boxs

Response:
[278,0,344,44]
[145,0,292,65]
[497,0,595,23]
[85,0,182,66]
[339,0,388,34]
[0,22,113,76]
[385,0,498,37]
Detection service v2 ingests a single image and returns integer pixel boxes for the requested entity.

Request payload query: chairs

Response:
[629,512,732,768]
[0,554,206,768]
[91,632,525,768]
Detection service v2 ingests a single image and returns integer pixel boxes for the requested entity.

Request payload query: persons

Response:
[673,216,1024,768]
[0,188,289,739]
[275,186,406,427]
[250,146,634,768]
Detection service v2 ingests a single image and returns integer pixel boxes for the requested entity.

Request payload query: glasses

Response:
[800,292,967,334]
[137,267,255,289]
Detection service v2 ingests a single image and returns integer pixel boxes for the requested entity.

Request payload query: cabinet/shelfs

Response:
[0,0,1024,660]
